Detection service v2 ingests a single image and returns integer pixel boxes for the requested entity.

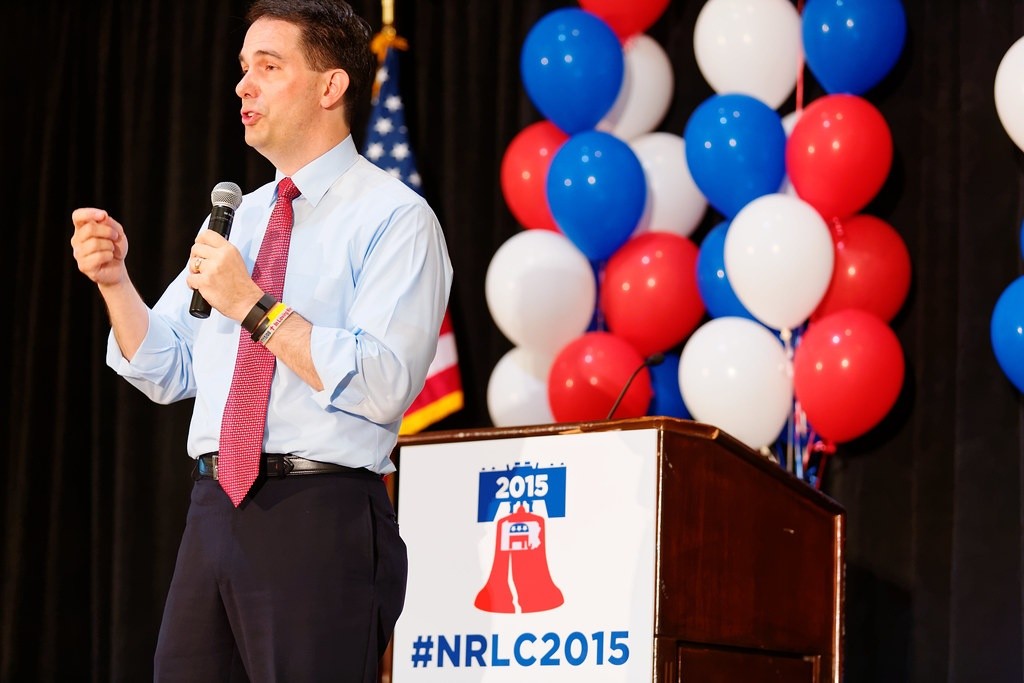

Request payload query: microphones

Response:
[606,354,666,421]
[189,181,242,318]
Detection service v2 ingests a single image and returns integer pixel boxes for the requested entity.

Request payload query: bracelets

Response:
[240,291,294,346]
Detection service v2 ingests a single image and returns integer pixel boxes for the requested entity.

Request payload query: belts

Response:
[199,455,348,481]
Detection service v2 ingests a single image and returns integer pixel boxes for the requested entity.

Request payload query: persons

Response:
[70,0,453,683]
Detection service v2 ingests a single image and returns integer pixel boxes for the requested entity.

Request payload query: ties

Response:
[219,177,301,507]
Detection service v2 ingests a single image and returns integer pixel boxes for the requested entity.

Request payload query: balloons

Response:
[993,37,1024,152]
[485,0,912,451]
[990,275,1024,393]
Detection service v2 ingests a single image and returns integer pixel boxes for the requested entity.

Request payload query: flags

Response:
[775,0,836,491]
[361,0,464,436]
